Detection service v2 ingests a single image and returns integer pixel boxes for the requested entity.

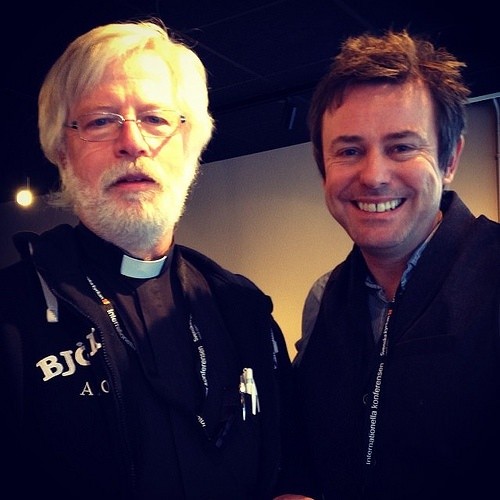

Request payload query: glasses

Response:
[64,109,186,142]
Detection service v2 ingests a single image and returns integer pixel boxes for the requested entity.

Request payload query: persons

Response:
[0,19,311,500]
[292,34,500,500]
